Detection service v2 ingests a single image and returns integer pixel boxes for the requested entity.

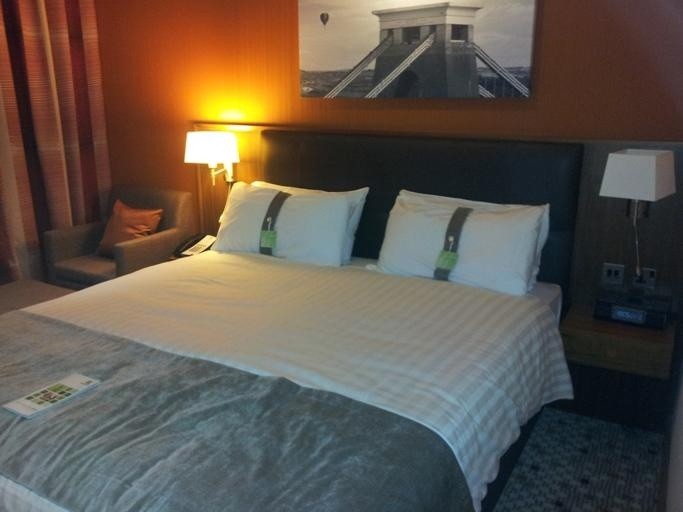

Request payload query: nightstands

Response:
[559,300,679,382]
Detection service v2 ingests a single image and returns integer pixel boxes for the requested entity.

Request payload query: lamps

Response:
[597,147,677,278]
[181,131,241,186]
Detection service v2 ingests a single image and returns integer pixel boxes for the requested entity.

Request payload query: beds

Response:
[0,125,586,512]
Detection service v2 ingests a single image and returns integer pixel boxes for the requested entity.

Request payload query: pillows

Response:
[212,180,370,270]
[365,189,551,297]
[93,198,165,260]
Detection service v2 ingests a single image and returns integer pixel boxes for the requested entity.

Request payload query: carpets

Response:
[485,404,670,511]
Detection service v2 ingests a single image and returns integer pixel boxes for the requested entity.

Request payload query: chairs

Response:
[38,183,194,286]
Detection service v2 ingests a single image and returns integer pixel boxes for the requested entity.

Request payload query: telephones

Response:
[175,233,217,257]
